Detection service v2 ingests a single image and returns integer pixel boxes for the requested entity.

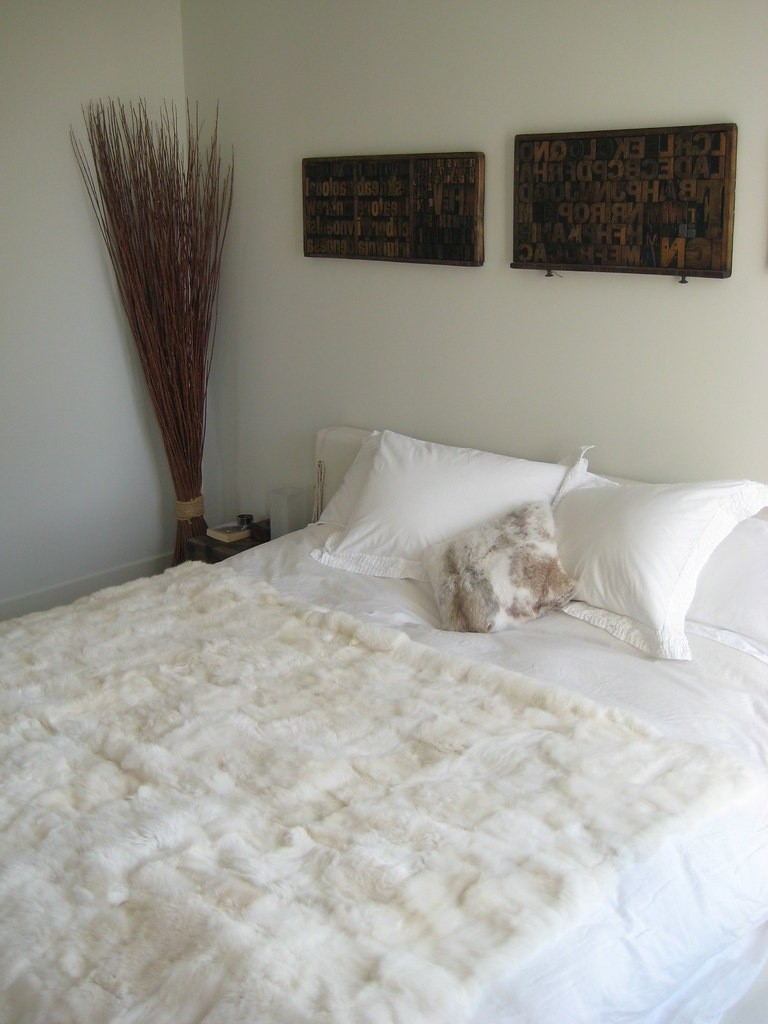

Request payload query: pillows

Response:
[420,499,576,632]
[550,458,768,661]
[306,430,383,526]
[596,473,768,664]
[310,430,568,584]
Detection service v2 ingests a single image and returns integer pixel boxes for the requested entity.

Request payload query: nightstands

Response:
[186,524,270,564]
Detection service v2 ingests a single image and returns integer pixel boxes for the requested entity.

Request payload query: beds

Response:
[0,425,767,1024]
